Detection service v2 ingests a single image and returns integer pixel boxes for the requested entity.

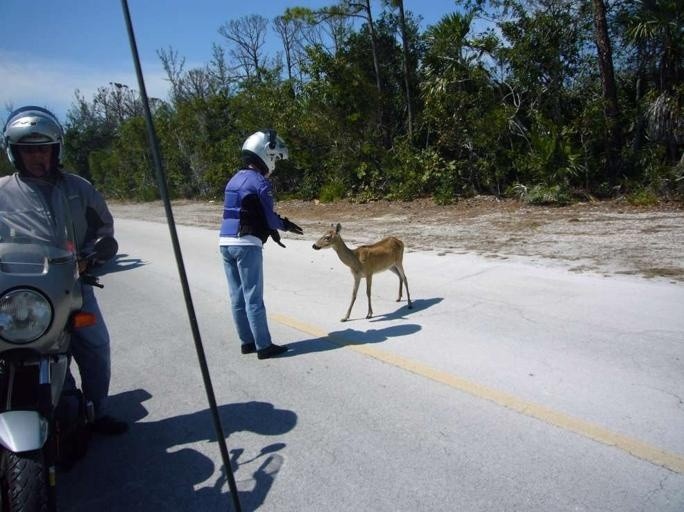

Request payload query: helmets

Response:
[242,128,289,177]
[0,106,69,172]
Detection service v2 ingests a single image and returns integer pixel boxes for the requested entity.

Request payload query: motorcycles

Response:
[1,173,120,511]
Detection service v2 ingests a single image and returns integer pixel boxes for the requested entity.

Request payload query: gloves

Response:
[271,230,286,247]
[284,217,303,235]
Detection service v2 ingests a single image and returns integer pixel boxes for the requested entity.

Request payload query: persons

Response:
[0,105,129,434]
[220,131,303,359]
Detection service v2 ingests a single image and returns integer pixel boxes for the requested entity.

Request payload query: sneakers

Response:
[94,412,129,440]
[258,343,288,358]
[241,343,256,352]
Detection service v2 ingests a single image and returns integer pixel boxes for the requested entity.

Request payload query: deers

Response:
[312,222,412,322]
